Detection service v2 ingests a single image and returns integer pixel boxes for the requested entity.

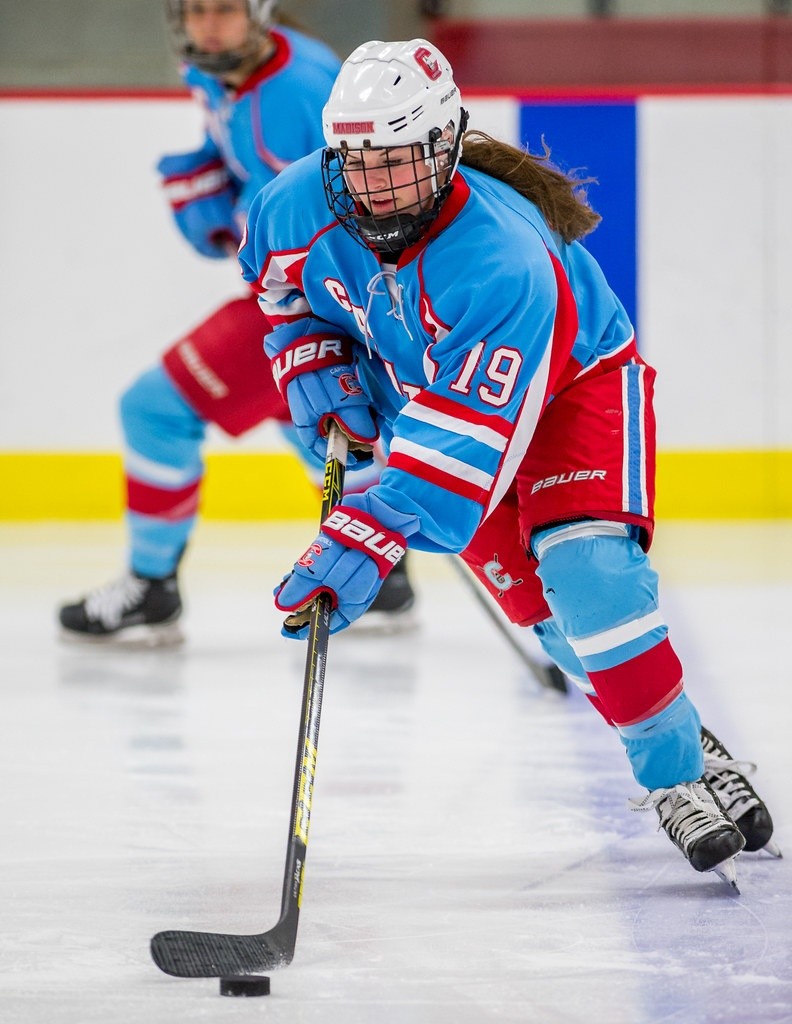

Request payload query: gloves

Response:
[263,317,379,471]
[157,129,245,260]
[274,492,420,640]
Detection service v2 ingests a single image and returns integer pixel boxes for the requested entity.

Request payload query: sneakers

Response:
[629,774,745,895]
[58,543,188,642]
[367,553,416,615]
[697,724,784,860]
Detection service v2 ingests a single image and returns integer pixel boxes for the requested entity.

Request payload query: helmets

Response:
[321,37,470,252]
[166,0,268,74]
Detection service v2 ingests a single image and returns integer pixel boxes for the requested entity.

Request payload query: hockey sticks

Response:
[219,236,571,695]
[148,416,349,979]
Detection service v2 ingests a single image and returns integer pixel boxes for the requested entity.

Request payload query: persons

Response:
[60,0,418,649]
[237,40,785,899]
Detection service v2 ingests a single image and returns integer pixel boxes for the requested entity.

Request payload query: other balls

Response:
[218,975,270,996]
